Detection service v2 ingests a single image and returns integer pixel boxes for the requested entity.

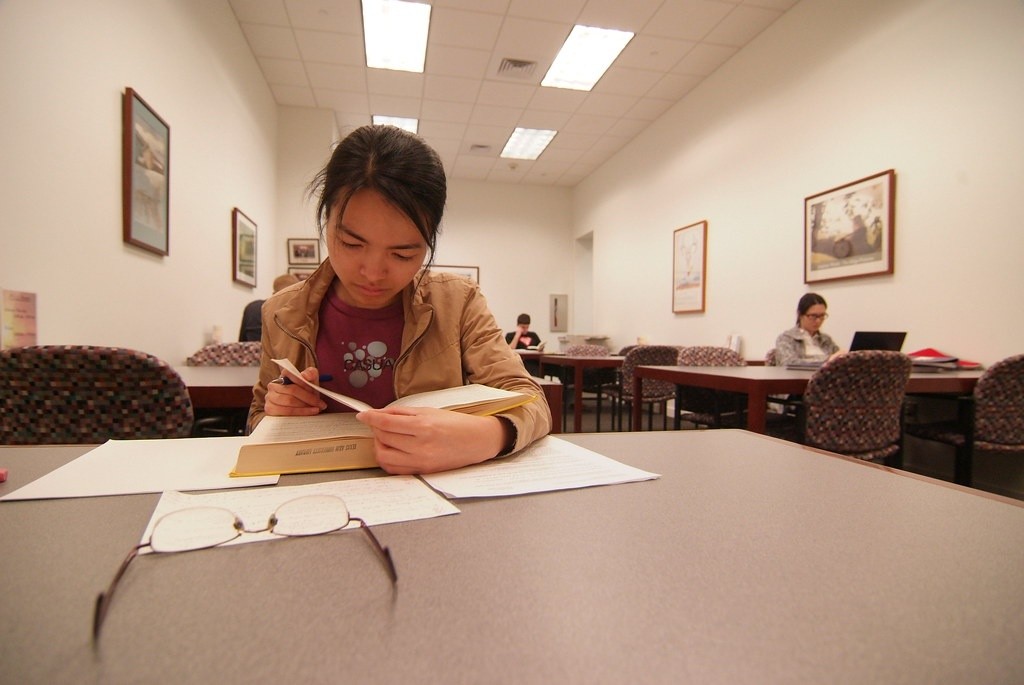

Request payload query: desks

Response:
[538,355,765,433]
[514,348,618,362]
[172,365,260,409]
[632,364,984,435]
[0,427,1024,685]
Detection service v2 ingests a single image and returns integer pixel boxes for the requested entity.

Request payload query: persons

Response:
[243,274,300,342]
[776,293,842,367]
[506,314,545,352]
[245,124,553,474]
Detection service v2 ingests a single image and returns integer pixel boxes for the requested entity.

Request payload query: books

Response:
[230,384,535,477]
[907,348,981,373]
[527,339,546,350]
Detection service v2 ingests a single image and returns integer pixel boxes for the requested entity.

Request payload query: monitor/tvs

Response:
[849,332,907,352]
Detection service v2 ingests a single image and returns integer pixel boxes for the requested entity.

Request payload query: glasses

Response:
[803,313,828,321]
[93,494,397,639]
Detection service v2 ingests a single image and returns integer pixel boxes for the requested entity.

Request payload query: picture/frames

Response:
[803,168,895,285]
[123,87,172,256]
[421,265,479,285]
[232,207,258,288]
[671,220,708,315]
[287,238,321,275]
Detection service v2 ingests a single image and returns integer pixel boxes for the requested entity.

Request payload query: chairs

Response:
[0,344,195,445]
[560,344,616,433]
[903,355,1024,488]
[769,350,913,468]
[186,340,262,438]
[674,345,748,430]
[596,346,679,432]
